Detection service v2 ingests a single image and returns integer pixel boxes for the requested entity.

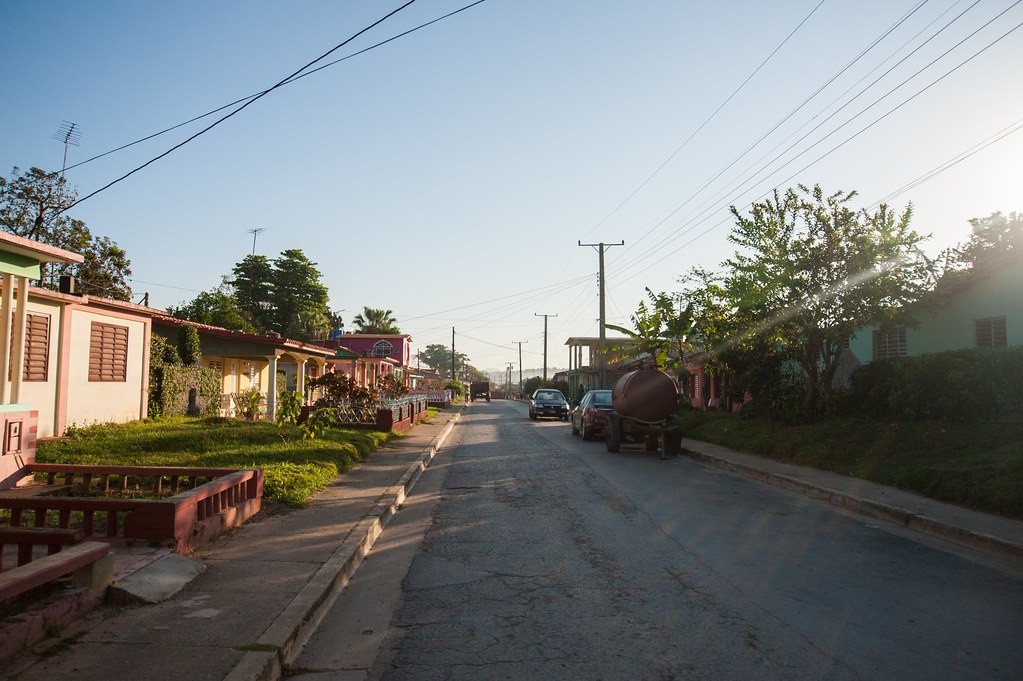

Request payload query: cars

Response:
[570,389,613,440]
[528,389,571,422]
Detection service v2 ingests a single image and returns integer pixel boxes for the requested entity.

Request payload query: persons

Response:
[577,383,584,401]
[465,386,470,401]
